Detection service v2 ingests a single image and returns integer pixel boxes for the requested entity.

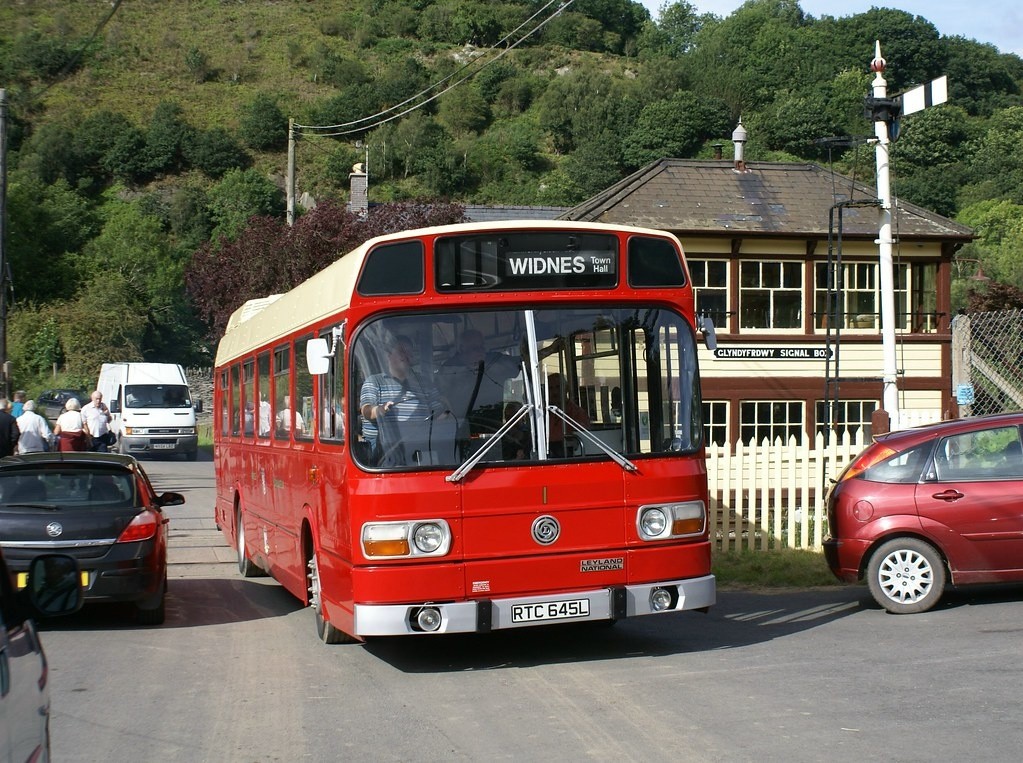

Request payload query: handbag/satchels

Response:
[106,430,117,445]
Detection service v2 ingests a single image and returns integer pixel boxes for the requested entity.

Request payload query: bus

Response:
[212,220,734,646]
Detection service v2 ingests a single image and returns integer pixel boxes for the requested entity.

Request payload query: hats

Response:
[23,400,35,410]
[14,390,26,399]
[65,398,81,410]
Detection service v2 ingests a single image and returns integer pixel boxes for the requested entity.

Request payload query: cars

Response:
[36,388,92,421]
[0,549,85,763]
[0,450,186,627]
[821,412,1023,615]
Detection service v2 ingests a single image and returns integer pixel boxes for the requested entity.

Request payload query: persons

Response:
[235,389,345,441]
[0,390,113,457]
[361,334,447,463]
[443,330,589,458]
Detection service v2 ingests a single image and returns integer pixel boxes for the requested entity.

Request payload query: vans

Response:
[97,361,202,462]
